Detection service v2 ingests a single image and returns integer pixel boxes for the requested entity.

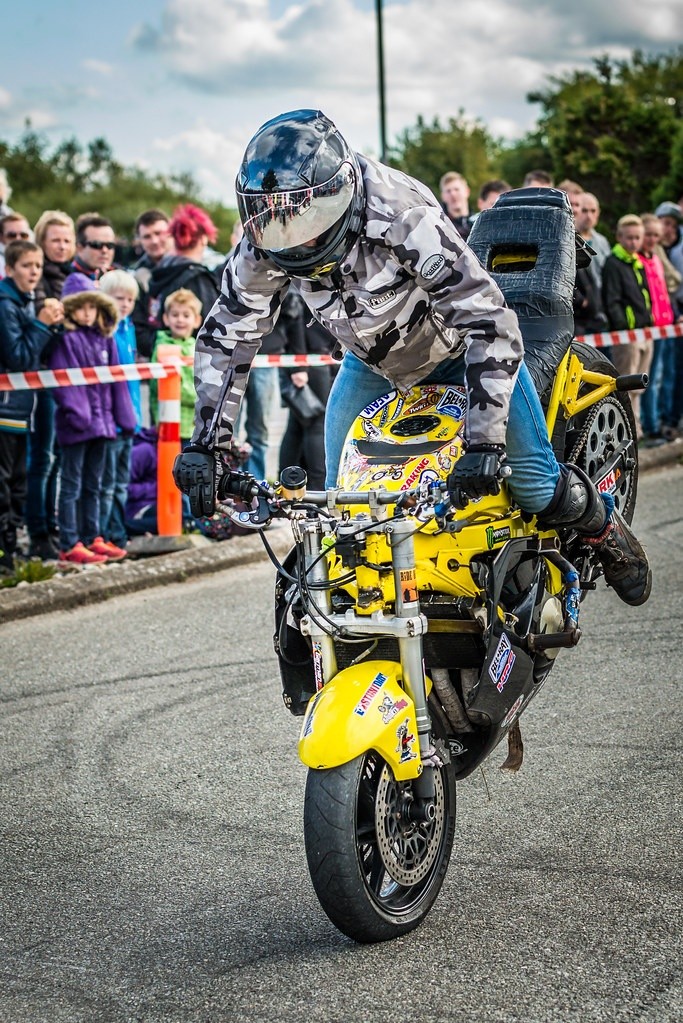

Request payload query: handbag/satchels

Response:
[281,383,325,430]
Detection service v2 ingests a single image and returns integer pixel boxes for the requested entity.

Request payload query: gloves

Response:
[174,445,231,519]
[450,444,508,509]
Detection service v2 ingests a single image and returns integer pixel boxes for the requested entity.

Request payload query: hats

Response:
[655,202,683,225]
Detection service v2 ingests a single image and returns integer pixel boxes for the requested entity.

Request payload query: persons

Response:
[1,169,682,575]
[172,110,652,607]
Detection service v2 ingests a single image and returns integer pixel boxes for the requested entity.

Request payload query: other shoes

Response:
[0,536,126,575]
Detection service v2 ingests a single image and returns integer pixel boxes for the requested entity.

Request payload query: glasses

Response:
[81,240,117,249]
[1,232,28,239]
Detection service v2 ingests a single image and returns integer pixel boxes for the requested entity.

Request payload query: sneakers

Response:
[536,463,652,606]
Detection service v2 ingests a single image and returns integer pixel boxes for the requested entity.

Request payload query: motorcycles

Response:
[168,189,662,950]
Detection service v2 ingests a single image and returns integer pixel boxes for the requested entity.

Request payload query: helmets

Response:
[236,109,365,282]
